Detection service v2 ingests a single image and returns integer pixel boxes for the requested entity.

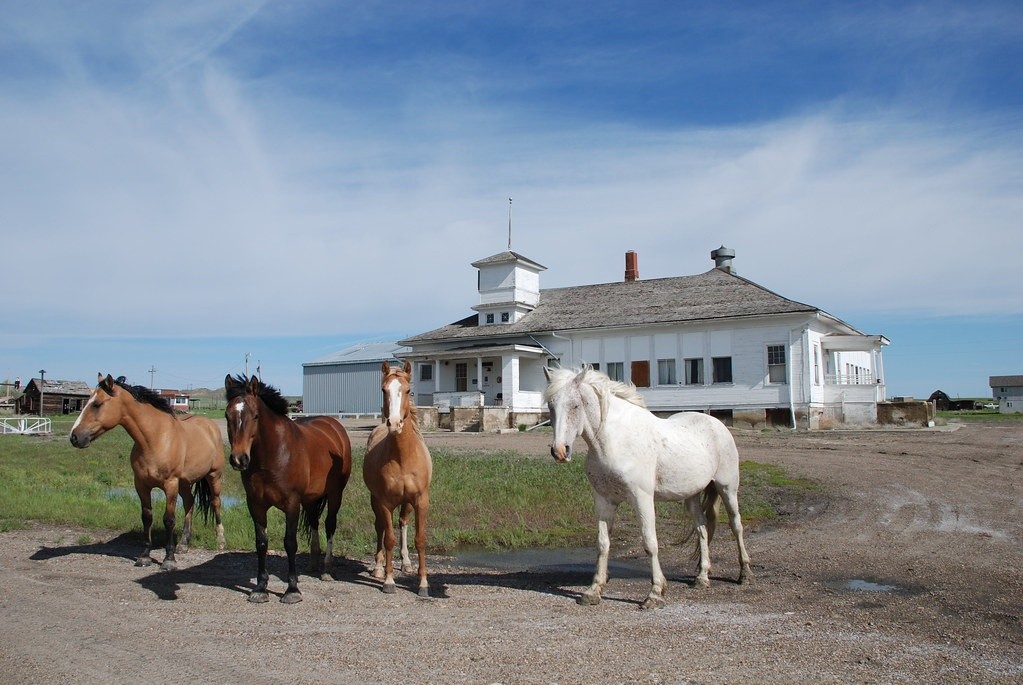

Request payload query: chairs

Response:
[493,393,502,405]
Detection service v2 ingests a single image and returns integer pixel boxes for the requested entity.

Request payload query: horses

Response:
[68,373,227,574]
[541,365,757,611]
[361,358,434,598]
[224,373,352,605]
